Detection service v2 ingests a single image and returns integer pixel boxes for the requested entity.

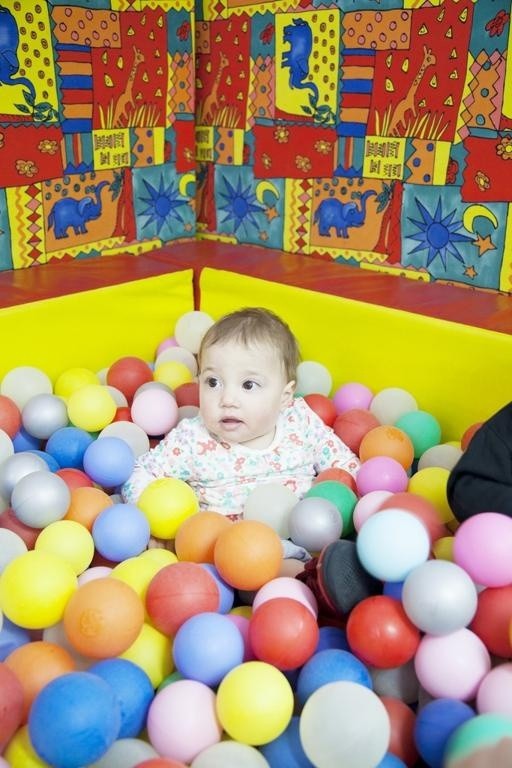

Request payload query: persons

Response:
[447,400,511,524]
[122,306,384,611]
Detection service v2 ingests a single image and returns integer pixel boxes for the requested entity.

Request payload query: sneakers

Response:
[295,540,384,625]
